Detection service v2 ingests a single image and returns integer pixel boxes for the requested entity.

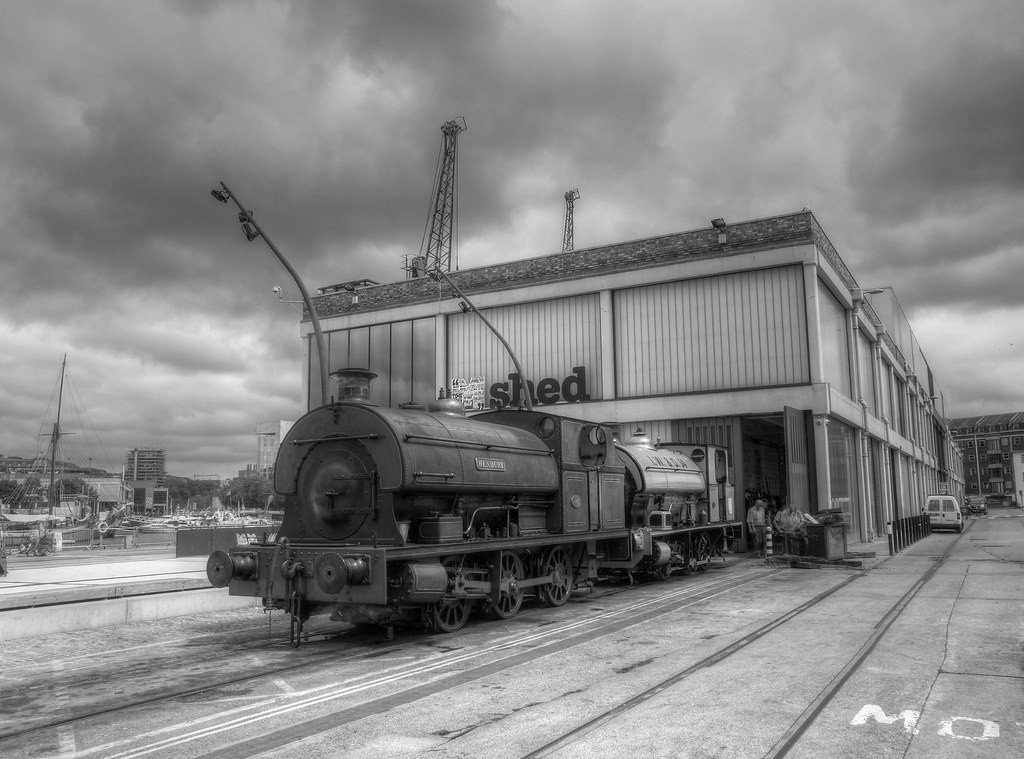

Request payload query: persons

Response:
[747,499,771,559]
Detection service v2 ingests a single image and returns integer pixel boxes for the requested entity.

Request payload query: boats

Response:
[121,495,280,534]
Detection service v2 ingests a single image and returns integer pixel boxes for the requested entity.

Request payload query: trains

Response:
[203,181,745,650]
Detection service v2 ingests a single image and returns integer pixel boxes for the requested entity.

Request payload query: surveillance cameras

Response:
[272,286,278,293]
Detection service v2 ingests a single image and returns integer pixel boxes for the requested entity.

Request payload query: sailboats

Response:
[0,351,136,548]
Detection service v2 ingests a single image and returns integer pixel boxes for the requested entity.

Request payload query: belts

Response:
[755,525,764,527]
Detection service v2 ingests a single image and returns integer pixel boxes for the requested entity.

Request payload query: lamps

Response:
[344,283,359,295]
[711,217,727,233]
[458,301,472,313]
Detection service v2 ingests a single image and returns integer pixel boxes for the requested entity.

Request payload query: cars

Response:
[967,496,989,514]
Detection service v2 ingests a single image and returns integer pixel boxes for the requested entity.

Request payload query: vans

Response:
[920,494,965,534]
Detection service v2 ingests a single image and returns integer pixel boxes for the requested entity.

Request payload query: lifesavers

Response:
[97,522,108,533]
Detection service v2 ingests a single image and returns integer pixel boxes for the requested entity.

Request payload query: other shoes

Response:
[756,553,764,558]
